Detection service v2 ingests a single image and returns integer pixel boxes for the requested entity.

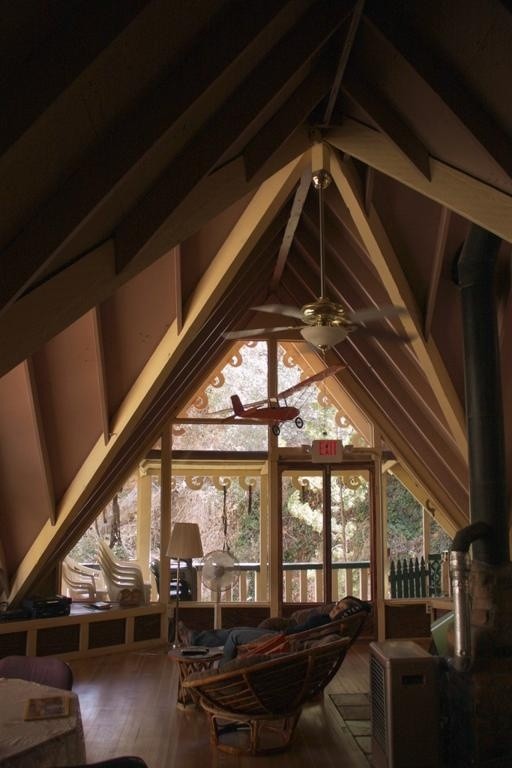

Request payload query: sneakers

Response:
[176,620,191,645]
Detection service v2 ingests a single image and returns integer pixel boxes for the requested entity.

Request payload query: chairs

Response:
[61,537,193,605]
[0,655,74,692]
[170,593,375,755]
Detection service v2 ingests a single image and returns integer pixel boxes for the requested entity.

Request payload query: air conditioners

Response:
[369,640,437,768]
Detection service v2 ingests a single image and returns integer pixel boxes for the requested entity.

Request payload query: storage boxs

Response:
[29,596,75,619]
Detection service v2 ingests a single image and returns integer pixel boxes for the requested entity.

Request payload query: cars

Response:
[76,563,108,598]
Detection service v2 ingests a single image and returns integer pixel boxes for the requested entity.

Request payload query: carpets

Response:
[330,688,371,767]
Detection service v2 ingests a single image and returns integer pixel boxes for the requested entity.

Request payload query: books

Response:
[93,600,112,609]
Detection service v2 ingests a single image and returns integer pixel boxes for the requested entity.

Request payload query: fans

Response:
[219,168,418,357]
[199,548,242,631]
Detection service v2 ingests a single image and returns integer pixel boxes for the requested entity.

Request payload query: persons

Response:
[178,597,360,713]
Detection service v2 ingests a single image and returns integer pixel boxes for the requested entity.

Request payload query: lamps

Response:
[166,521,203,650]
[299,324,346,354]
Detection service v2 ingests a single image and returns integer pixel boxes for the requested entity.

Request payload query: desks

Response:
[0,676,88,767]
